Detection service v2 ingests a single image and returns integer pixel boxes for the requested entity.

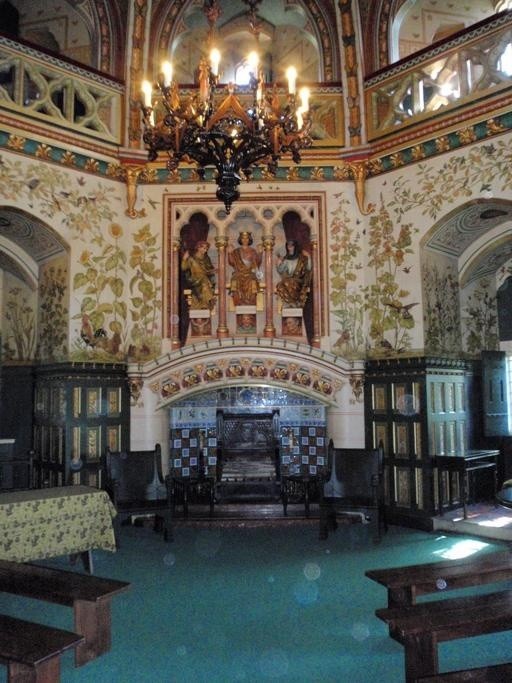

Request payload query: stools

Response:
[279,472,322,517]
[171,473,214,517]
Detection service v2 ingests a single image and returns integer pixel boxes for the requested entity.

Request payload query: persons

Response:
[275,240,310,307]
[181,240,216,306]
[228,231,260,305]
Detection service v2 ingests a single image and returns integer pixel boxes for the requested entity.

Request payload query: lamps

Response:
[137,0,316,215]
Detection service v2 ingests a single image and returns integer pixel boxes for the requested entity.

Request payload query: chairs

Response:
[213,406,284,505]
[316,435,390,545]
[103,441,175,549]
[0,449,36,491]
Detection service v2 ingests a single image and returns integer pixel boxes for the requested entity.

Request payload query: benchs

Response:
[0,612,87,682]
[372,586,512,683]
[1,555,132,668]
[361,548,511,648]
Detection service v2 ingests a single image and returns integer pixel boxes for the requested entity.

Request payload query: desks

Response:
[1,482,119,576]
[433,446,502,520]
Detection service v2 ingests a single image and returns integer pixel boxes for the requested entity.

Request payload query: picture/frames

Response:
[161,191,328,343]
